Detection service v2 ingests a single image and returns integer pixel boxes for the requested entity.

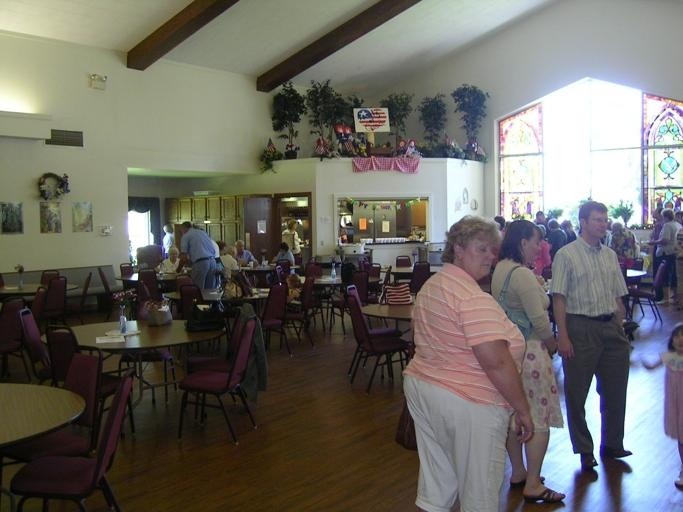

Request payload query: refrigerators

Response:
[352,200,397,244]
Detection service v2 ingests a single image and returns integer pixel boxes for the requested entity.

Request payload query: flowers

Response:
[258,144,281,174]
[38,173,70,201]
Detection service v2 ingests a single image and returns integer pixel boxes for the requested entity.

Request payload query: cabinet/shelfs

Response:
[164,193,271,248]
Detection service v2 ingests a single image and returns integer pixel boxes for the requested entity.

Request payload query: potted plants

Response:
[269,77,491,161]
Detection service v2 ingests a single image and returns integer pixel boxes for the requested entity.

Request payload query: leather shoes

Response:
[599,449,631,459]
[580,454,598,470]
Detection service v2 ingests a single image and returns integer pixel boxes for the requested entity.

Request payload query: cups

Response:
[249,262,253,268]
[360,237,406,243]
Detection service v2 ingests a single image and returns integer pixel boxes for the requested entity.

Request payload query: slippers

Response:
[523,488,565,504]
[509,475,545,489]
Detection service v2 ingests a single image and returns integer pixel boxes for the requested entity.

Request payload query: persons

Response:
[490,206,682,302]
[640,320,683,490]
[399,214,534,512]
[550,200,633,471]
[488,218,566,503]
[153,220,304,309]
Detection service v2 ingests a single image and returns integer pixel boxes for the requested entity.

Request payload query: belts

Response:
[594,313,611,321]
[195,257,209,263]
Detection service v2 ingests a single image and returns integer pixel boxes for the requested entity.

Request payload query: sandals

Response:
[658,281,681,311]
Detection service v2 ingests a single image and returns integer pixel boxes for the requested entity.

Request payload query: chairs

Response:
[9,371,135,512]
[260,244,446,397]
[475,252,664,337]
[0,245,267,460]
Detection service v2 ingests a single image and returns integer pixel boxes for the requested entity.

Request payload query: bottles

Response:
[330,263,337,281]
[125,319,137,332]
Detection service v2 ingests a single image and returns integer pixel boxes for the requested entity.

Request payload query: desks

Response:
[0,381,87,510]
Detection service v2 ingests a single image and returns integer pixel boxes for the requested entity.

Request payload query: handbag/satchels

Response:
[222,277,243,301]
[183,297,226,333]
[498,267,530,339]
[384,282,413,305]
[231,270,256,297]
[396,400,417,449]
[144,298,173,326]
